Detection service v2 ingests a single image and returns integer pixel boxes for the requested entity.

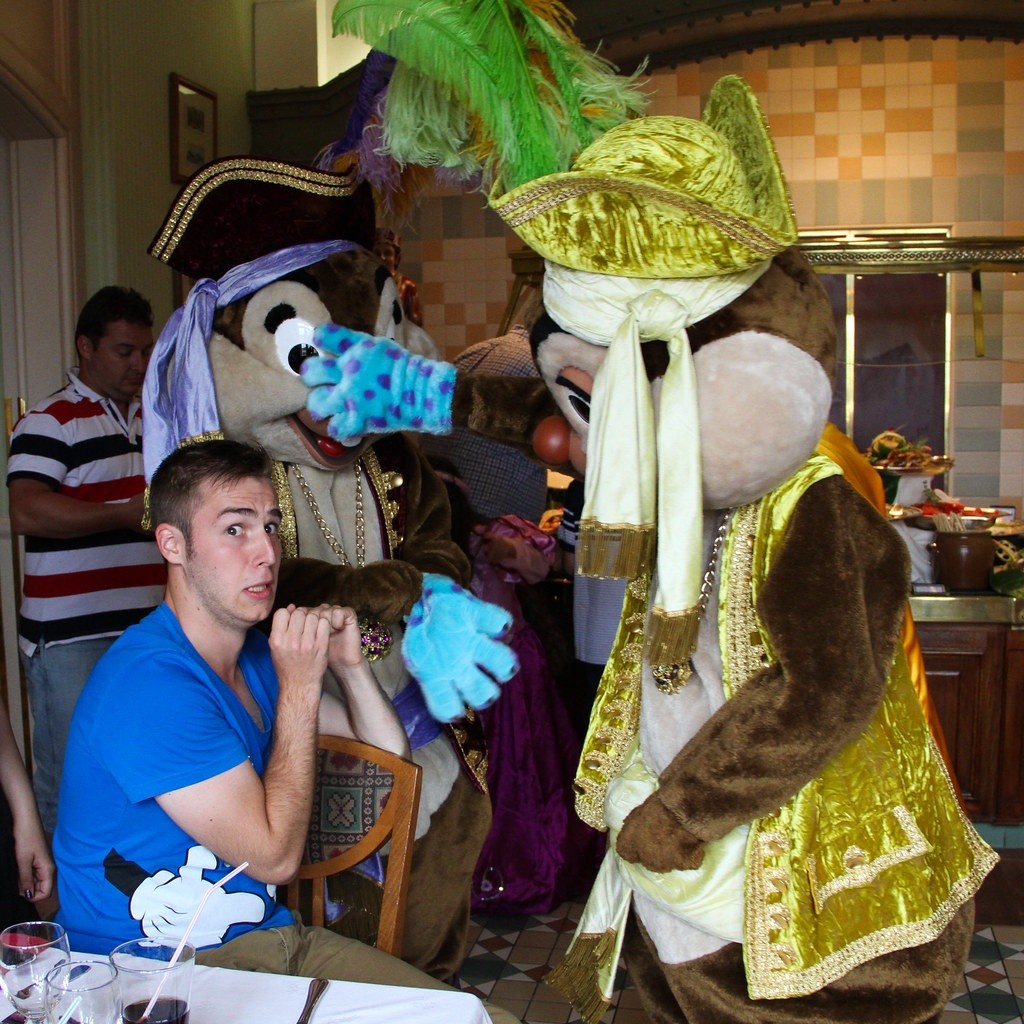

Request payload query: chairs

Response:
[275,734,421,961]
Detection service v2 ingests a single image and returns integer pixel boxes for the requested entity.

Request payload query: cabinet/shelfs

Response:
[907,621,1024,851]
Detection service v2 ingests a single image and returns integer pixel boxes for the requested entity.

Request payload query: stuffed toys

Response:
[142,91,583,983]
[298,0,1003,1023]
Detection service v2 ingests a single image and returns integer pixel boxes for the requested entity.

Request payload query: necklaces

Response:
[292,457,394,663]
[640,511,729,694]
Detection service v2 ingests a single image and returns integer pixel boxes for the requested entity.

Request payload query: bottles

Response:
[926,531,999,595]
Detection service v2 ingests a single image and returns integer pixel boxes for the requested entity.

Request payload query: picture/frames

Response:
[167,72,219,184]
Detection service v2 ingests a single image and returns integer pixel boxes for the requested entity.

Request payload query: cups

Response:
[0,923,71,1024]
[44,960,121,1024]
[109,936,196,1024]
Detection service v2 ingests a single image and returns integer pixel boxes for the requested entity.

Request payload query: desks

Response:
[0,945,497,1024]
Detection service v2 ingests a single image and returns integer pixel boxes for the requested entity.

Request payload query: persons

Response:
[8,285,167,937]
[56,441,455,987]
[0,699,58,946]
